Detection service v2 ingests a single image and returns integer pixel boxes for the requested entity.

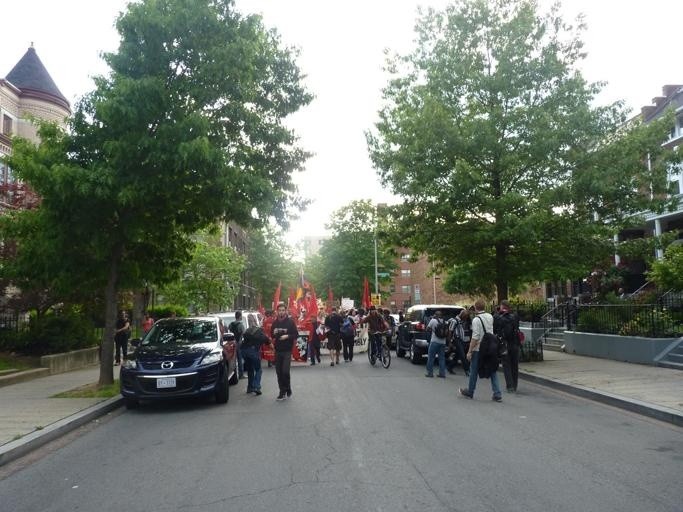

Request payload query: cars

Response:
[119,314,238,410]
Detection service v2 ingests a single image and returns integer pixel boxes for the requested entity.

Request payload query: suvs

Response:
[395,300,471,367]
[206,310,263,336]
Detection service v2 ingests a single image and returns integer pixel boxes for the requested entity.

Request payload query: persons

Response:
[228,301,520,402]
[114,310,179,366]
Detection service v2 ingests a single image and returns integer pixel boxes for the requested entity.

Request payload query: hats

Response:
[369,306,377,310]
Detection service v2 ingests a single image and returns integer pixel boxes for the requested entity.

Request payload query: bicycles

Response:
[364,329,394,371]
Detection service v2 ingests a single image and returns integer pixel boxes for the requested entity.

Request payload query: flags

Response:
[257,263,372,330]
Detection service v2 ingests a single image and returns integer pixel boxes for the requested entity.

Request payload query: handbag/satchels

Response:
[451,328,461,346]
[241,345,259,361]
[499,334,508,357]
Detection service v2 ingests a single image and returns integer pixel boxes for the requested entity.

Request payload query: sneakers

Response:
[459,388,473,399]
[492,396,502,402]
[311,357,339,366]
[115,363,120,366]
[277,390,292,400]
[425,368,469,378]
[246,387,262,395]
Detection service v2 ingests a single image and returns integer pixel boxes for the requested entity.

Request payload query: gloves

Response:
[388,330,392,336]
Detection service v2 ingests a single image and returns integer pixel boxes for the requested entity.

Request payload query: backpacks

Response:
[340,318,352,332]
[433,318,449,338]
